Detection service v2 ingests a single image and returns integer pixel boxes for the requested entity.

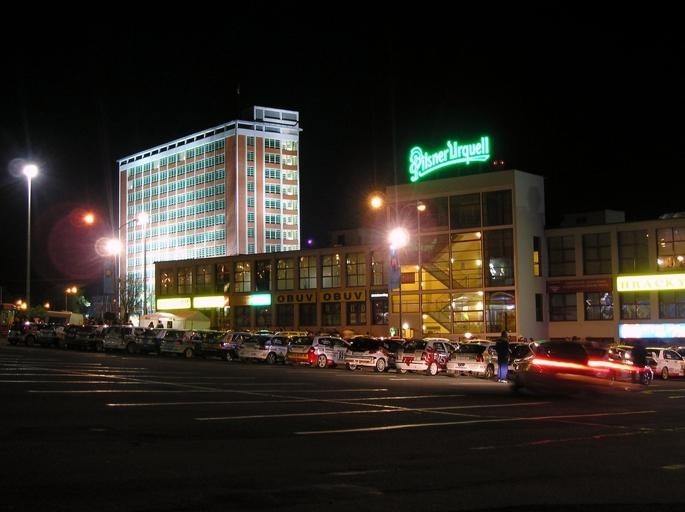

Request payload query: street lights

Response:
[23,164,38,308]
[84,212,150,316]
[370,195,427,339]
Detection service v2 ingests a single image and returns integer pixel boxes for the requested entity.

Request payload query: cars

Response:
[507,340,684,390]
[394,337,457,376]
[7,321,349,370]
[343,339,403,374]
[446,343,500,380]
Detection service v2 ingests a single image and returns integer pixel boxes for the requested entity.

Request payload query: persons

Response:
[145,320,157,328]
[508,334,584,344]
[630,340,646,383]
[495,330,513,383]
[155,319,165,328]
